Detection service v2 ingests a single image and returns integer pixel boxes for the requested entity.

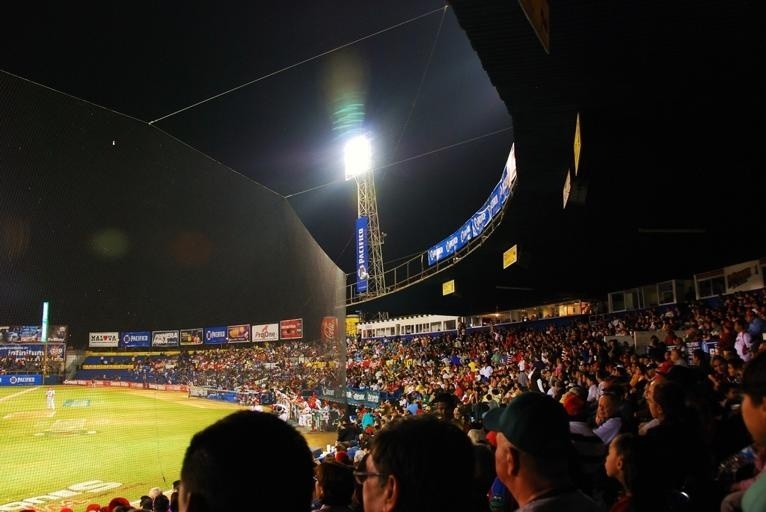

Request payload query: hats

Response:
[483,392,570,454]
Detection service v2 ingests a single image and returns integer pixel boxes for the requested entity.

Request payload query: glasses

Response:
[353,454,390,484]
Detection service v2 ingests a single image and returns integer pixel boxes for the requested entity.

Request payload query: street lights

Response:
[341,130,388,300]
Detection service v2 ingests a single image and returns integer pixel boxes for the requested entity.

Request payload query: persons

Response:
[85,480,178,511]
[179,287,765,510]
[44,387,55,416]
[0,350,177,384]
